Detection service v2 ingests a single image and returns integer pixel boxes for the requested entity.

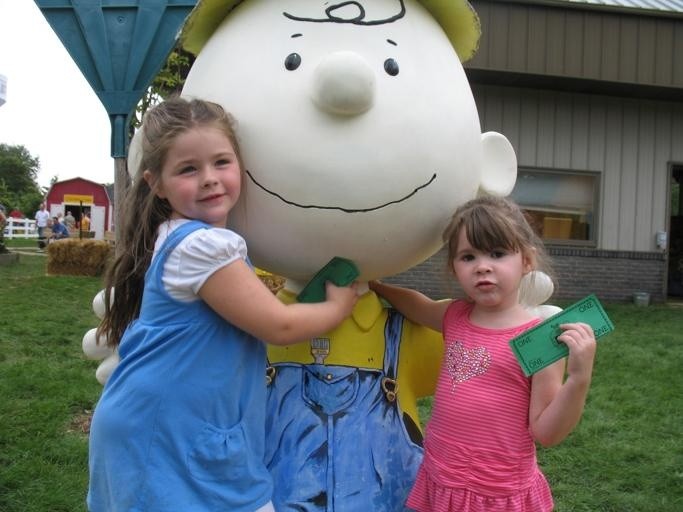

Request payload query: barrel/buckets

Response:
[633,291,649,309]
[571,220,588,240]
[80,230,94,238]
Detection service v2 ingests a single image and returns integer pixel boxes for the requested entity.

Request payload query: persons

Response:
[86,94,359,511]
[366,191,596,512]
[0,202,89,254]
